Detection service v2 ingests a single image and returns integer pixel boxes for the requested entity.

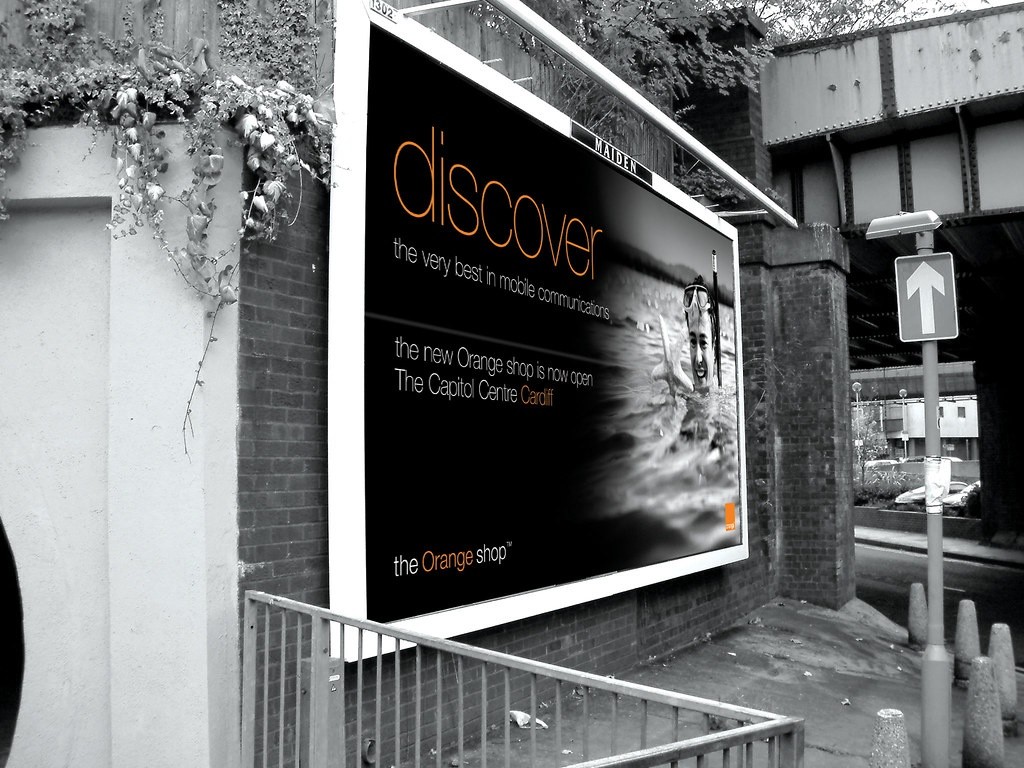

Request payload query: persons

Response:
[682,275,717,391]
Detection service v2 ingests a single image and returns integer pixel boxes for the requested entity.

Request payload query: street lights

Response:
[852,383,862,465]
[863,211,956,768]
[898,388,910,460]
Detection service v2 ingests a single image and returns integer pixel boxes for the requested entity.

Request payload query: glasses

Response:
[682,285,712,312]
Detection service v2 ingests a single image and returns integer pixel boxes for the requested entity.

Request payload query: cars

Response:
[942,481,981,517]
[892,481,968,512]
